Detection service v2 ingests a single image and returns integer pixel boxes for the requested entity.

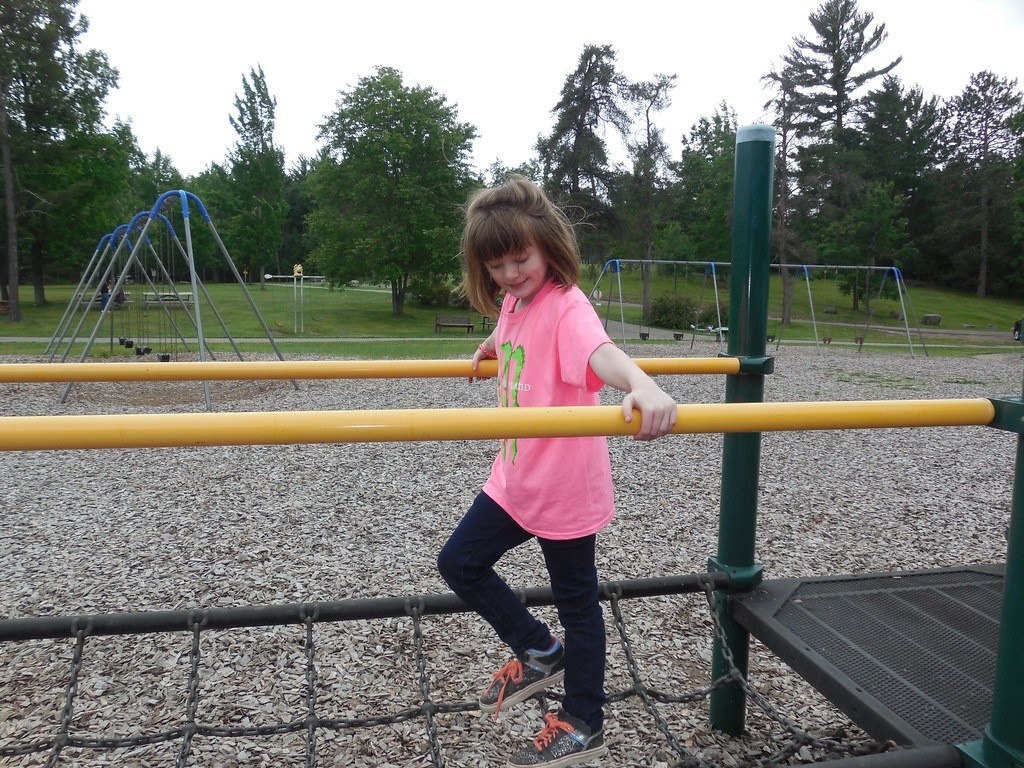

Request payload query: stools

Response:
[715,326,729,342]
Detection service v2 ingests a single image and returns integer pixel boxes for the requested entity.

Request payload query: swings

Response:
[639,261,651,340]
[309,278,324,321]
[118,201,179,362]
[854,268,870,344]
[823,267,837,345]
[767,266,779,343]
[272,276,288,328]
[673,262,688,341]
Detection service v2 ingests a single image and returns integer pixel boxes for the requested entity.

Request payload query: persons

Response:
[97,272,112,313]
[435,174,679,767]
[114,287,125,308]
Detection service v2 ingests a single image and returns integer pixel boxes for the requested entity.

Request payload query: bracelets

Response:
[478,343,497,360]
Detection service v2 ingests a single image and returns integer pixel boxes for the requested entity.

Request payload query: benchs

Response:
[435,315,474,334]
[78,292,133,312]
[143,292,194,310]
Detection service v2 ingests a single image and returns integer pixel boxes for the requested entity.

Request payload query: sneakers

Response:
[506,709,605,768]
[479,635,566,720]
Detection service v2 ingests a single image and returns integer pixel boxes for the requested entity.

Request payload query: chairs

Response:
[482,316,497,333]
